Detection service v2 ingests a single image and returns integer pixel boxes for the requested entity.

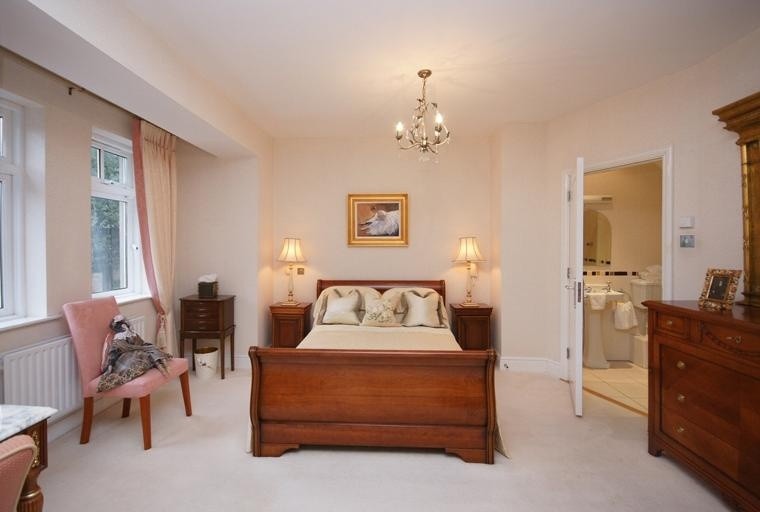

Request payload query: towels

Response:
[613,302,639,330]
[590,292,606,311]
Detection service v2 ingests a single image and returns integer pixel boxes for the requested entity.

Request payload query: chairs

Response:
[62,294,193,452]
[0,434,38,512]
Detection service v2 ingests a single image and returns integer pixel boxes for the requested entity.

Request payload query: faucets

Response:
[607,281,611,291]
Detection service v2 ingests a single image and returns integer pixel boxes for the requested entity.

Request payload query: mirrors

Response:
[583,209,613,268]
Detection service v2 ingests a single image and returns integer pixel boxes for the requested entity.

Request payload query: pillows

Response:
[401,290,444,328]
[322,288,360,325]
[357,287,402,327]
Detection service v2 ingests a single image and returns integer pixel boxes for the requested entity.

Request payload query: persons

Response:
[360,205,400,236]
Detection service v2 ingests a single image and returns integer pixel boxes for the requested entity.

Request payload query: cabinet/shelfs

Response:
[178,295,237,380]
[641,298,760,512]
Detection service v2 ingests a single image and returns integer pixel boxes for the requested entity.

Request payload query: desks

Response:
[0,403,59,512]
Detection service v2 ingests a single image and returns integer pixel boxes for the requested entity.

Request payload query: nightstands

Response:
[449,302,494,350]
[269,301,313,348]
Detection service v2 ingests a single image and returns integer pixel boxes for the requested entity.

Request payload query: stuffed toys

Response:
[96,315,174,394]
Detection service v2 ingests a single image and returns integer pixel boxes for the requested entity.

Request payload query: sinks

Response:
[588,290,623,300]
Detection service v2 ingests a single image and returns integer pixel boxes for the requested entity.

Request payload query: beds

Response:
[247,277,498,465]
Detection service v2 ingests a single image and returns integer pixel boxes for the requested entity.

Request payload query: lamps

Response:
[277,238,306,307]
[450,236,488,308]
[393,68,452,156]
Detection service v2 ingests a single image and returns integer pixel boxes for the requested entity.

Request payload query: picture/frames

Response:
[347,191,410,248]
[698,267,742,315]
